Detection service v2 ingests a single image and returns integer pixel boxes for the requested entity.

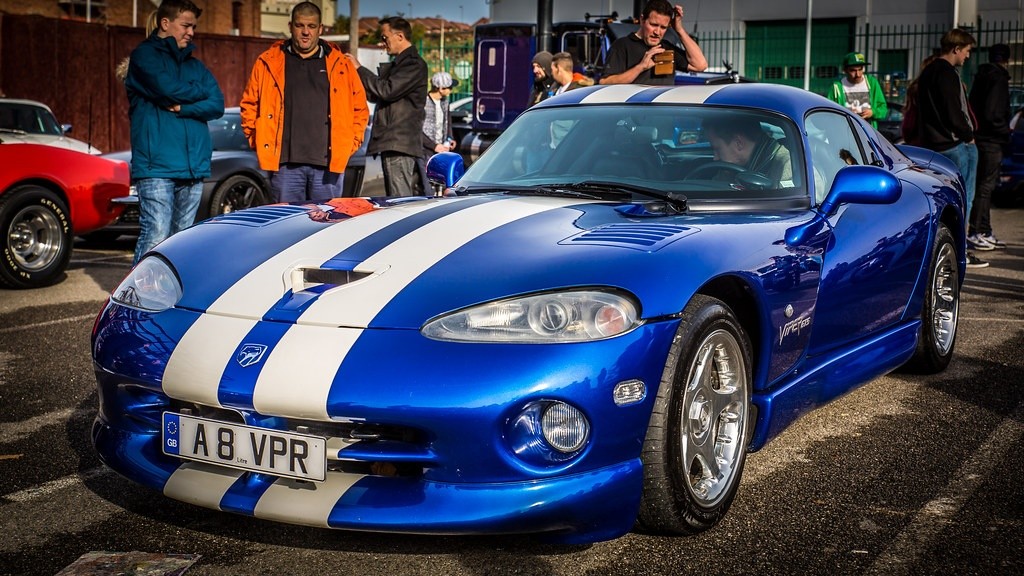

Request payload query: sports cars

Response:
[88,81,972,537]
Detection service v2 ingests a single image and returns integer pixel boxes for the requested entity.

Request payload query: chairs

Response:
[590,156,636,179]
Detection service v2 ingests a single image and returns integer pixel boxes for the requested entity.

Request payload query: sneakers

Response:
[964,253,990,269]
[982,232,1006,249]
[966,233,996,250]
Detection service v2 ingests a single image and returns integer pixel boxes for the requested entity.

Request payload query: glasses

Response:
[383,30,402,42]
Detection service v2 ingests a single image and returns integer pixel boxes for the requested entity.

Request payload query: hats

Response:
[843,51,872,67]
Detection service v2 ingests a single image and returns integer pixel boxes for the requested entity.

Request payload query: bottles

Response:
[547,91,553,98]
[443,137,452,148]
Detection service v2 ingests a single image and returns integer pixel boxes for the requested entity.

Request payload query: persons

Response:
[344,16,428,198]
[542,52,595,154]
[526,51,553,173]
[902,55,939,147]
[916,29,990,268]
[417,72,459,195]
[115,0,224,270]
[705,117,828,204]
[307,206,374,223]
[599,0,708,148]
[967,43,1012,250]
[825,53,889,164]
[239,0,369,204]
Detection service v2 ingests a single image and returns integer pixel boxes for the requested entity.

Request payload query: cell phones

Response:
[654,50,675,75]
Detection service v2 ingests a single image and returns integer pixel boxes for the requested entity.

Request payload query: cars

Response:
[0,96,74,138]
[0,125,131,290]
[448,97,502,168]
[79,105,385,244]
[877,85,1024,208]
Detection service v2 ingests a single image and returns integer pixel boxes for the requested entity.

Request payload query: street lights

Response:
[460,5,464,24]
[435,13,446,74]
[407,2,412,19]
